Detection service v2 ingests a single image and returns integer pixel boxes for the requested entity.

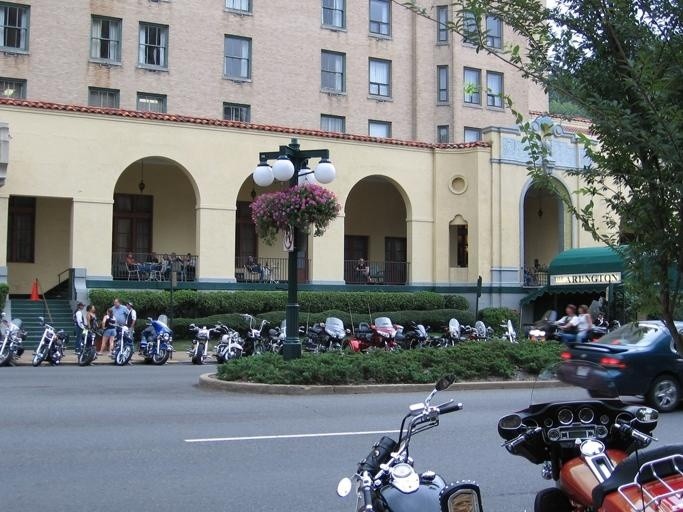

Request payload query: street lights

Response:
[250,136,337,364]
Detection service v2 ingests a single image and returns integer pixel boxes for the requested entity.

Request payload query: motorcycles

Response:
[31,317,69,365]
[336,370,485,512]
[527,318,611,347]
[189,314,287,365]
[495,354,682,512]
[306,315,520,348]
[137,314,177,366]
[75,325,106,366]
[1,313,30,366]
[108,319,137,365]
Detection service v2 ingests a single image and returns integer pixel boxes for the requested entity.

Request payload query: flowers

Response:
[249,185,341,248]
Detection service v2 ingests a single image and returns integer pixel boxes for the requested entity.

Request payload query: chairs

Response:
[243,264,262,284]
[125,261,167,282]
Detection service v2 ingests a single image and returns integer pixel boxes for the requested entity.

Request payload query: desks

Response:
[260,266,280,285]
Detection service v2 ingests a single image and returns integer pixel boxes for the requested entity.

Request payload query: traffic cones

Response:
[28,278,41,302]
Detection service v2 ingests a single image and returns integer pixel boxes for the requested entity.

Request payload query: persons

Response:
[96,308,116,357]
[124,301,136,338]
[110,298,129,334]
[125,251,144,278]
[553,304,577,344]
[246,256,264,283]
[86,304,98,345]
[355,256,371,282]
[73,302,86,355]
[593,312,609,327]
[163,252,178,281]
[575,304,592,344]
[182,253,195,266]
[153,253,170,272]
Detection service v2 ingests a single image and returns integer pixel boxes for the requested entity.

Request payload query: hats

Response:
[77,302,86,307]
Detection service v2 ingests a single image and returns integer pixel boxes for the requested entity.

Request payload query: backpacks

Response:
[106,315,116,329]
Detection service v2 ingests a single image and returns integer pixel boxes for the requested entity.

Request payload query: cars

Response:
[551,318,682,414]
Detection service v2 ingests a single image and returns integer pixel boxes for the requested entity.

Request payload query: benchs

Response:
[356,268,384,284]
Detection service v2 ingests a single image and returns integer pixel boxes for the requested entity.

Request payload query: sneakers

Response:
[96,352,103,355]
